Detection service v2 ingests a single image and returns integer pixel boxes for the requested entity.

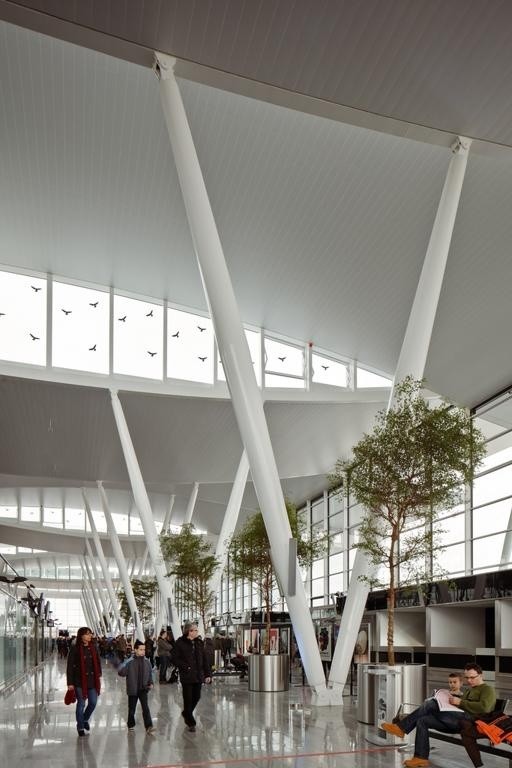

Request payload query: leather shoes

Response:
[159,681,169,684]
[182,711,197,732]
[84,722,89,730]
[381,723,404,738]
[403,757,430,767]
[78,730,85,737]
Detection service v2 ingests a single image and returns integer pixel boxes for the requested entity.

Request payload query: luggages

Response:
[167,666,179,683]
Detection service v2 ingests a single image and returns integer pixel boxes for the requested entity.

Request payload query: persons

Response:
[117,639,157,735]
[67,627,103,737]
[169,621,212,733]
[56,629,233,685]
[381,663,496,768]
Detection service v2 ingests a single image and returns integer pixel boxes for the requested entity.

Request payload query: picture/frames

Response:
[236,621,371,664]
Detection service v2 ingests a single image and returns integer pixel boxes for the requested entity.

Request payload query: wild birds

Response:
[148,351,157,357]
[278,357,286,362]
[61,309,72,315]
[197,326,206,332]
[0,313,6,317]
[198,356,207,362]
[171,331,179,338]
[89,344,96,351]
[118,316,126,322]
[31,285,42,292]
[321,365,329,371]
[89,301,99,308]
[29,333,40,341]
[146,309,154,317]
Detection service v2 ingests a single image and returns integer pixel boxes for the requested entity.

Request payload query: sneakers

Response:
[145,726,157,734]
[128,727,135,732]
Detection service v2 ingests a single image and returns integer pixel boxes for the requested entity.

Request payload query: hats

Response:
[64,690,76,705]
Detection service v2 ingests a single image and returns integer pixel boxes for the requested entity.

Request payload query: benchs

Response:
[391,665,512,768]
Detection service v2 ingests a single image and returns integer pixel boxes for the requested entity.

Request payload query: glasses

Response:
[464,674,480,679]
